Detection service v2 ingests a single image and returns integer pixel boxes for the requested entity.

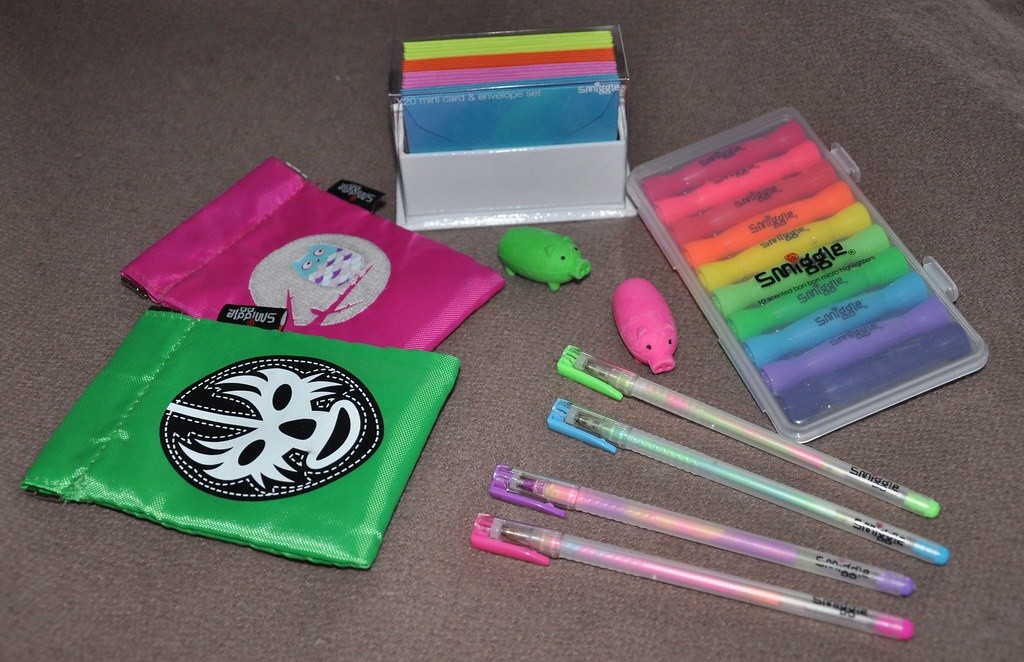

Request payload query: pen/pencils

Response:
[491,463,917,599]
[554,344,943,522]
[546,400,955,568]
[472,514,915,643]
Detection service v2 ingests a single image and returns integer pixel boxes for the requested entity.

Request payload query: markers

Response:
[638,119,980,422]
